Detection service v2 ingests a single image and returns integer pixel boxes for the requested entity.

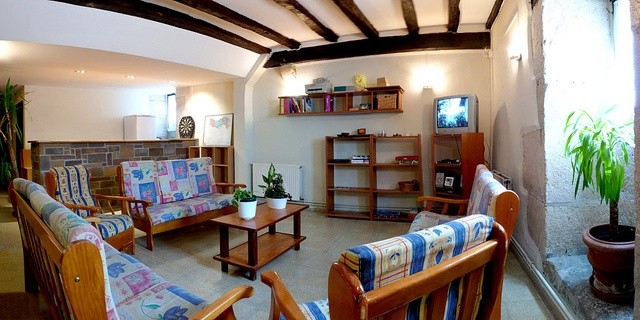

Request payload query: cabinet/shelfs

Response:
[277,90,404,115]
[374,134,423,222]
[324,136,373,220]
[432,132,485,215]
[187,147,233,192]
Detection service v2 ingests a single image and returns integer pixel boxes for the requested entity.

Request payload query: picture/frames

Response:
[202,113,234,146]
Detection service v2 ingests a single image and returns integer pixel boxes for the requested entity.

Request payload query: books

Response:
[350,155,371,165]
[281,96,313,115]
[396,155,418,164]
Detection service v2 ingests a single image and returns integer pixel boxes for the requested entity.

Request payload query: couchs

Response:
[8,177,254,320]
[262,214,508,319]
[117,156,247,250]
[409,165,520,251]
[46,164,136,258]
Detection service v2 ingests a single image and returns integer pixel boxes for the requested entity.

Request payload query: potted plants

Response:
[232,187,257,221]
[563,102,635,303]
[257,164,293,210]
[0,78,31,217]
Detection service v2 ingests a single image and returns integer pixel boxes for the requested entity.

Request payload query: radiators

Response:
[252,163,304,202]
[490,168,513,191]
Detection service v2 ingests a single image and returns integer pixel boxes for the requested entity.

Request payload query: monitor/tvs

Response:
[434,95,479,134]
